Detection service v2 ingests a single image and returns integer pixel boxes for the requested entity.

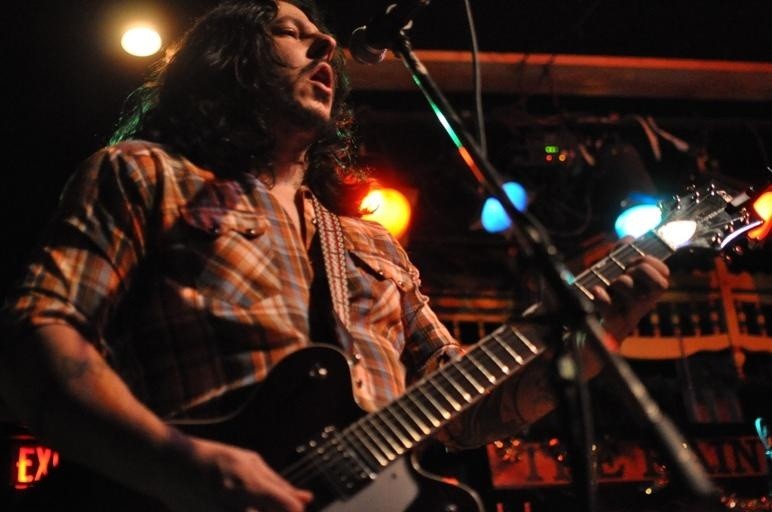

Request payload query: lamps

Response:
[599,145,663,241]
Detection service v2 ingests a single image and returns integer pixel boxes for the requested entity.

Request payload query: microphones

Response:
[348,0,429,68]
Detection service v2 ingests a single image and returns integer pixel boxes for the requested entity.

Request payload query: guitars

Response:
[0,184,764,512]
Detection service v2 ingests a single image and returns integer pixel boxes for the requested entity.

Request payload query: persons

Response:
[0,0,670,512]
